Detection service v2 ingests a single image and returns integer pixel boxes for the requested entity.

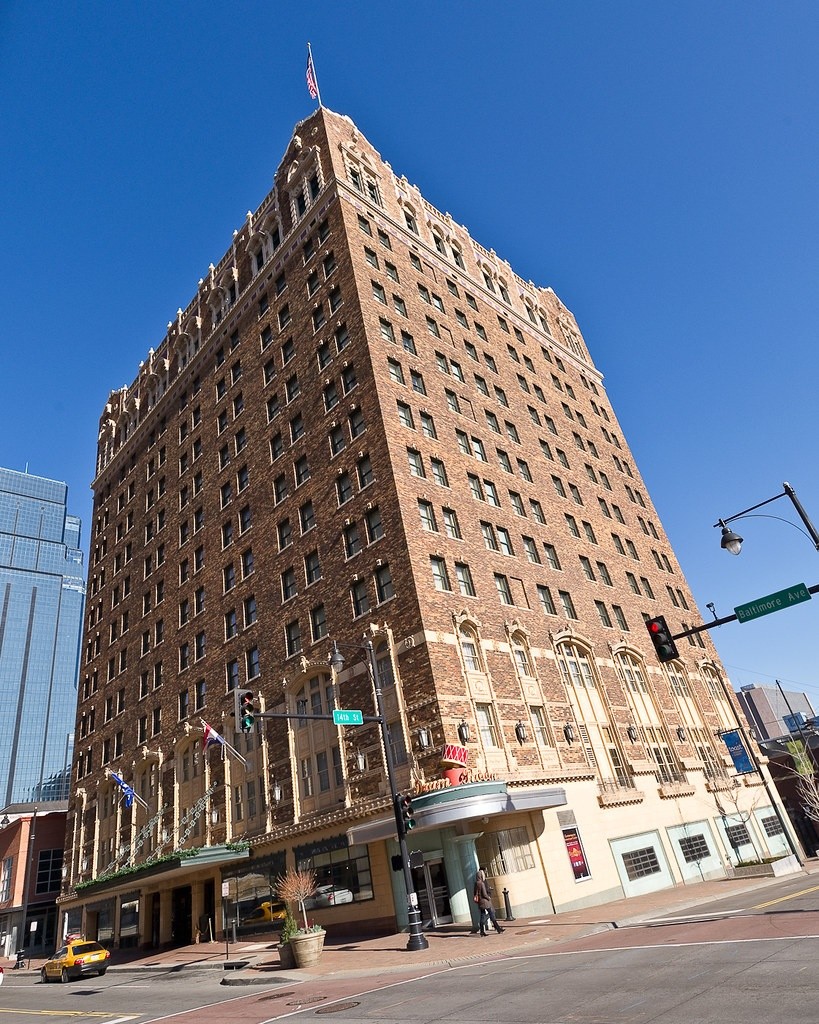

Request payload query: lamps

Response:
[62,858,67,880]
[119,836,126,862]
[676,724,686,741]
[515,719,527,744]
[749,727,756,739]
[272,780,281,805]
[210,804,217,826]
[354,746,364,774]
[563,722,574,743]
[459,717,470,742]
[417,724,429,749]
[161,818,168,845]
[81,850,88,875]
[718,727,724,740]
[627,723,637,742]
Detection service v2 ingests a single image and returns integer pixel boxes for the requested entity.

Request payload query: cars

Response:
[237,900,288,924]
[39,932,111,984]
[300,882,354,912]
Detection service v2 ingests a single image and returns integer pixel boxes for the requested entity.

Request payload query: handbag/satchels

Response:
[474,895,478,902]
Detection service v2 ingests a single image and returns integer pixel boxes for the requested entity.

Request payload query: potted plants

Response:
[276,902,301,968]
[269,859,326,969]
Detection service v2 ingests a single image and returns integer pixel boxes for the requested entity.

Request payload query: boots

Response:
[493,921,505,933]
[480,925,488,937]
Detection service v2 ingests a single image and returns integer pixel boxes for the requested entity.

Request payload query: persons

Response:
[65,935,73,944]
[475,867,505,937]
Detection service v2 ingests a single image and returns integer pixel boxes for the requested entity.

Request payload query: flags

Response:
[202,722,224,754]
[111,774,134,806]
[305,49,317,99]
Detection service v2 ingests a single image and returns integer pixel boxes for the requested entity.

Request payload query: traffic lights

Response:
[645,615,680,664]
[240,690,255,731]
